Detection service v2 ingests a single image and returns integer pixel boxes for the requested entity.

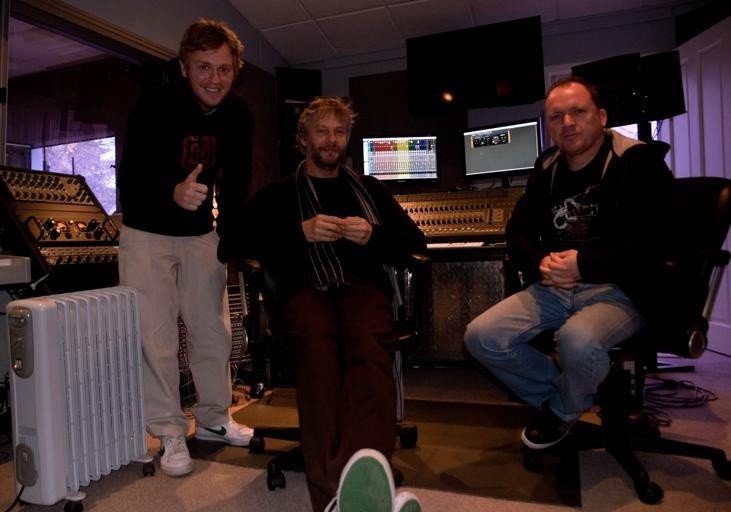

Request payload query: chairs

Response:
[243,251,432,491]
[522,177,731,506]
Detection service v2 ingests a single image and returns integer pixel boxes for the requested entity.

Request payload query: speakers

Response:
[275,66,322,105]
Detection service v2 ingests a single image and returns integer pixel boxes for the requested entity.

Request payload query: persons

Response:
[218,95,425,511]
[460,77,692,452]
[117,19,255,478]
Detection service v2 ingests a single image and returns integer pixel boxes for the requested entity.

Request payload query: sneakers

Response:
[195,420,254,446]
[521,404,579,449]
[159,436,192,476]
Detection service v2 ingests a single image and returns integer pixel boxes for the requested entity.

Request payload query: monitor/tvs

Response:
[360,134,440,194]
[462,116,544,188]
[29,136,116,216]
[406,15,545,116]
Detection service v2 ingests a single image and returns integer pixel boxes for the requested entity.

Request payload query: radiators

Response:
[6,286,155,511]
